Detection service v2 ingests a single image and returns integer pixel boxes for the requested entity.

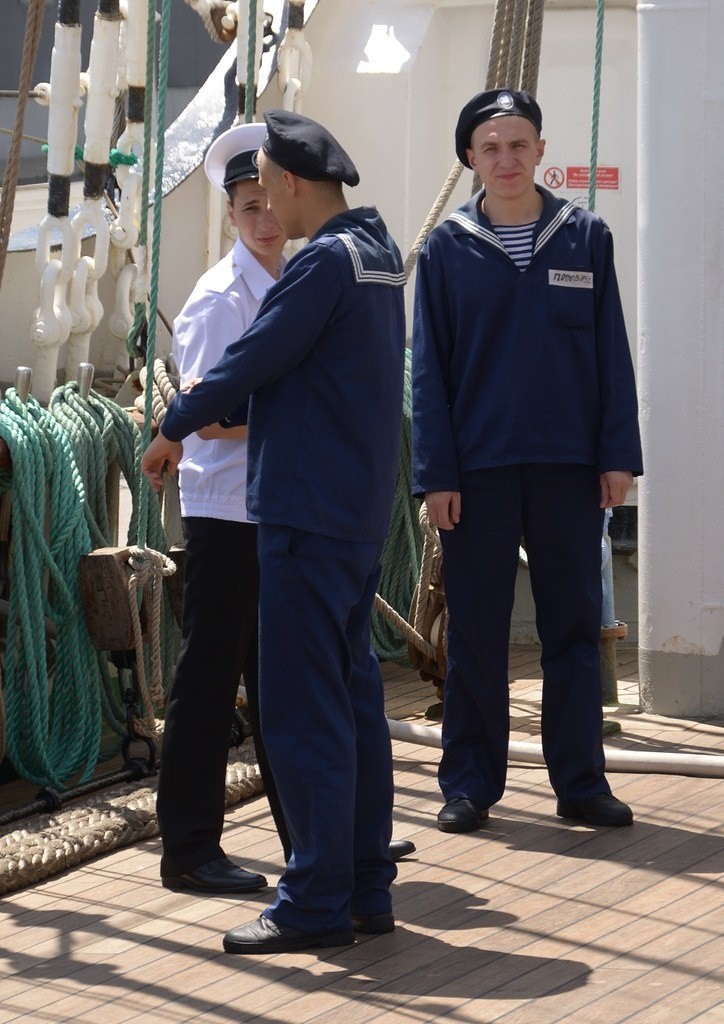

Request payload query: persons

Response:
[411,88,645,833]
[142,111,407,954]
[158,122,416,893]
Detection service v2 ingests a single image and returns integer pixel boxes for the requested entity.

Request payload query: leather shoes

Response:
[389,839,416,862]
[559,794,633,826]
[350,911,396,935]
[224,913,355,953]
[439,795,489,833]
[161,866,266,894]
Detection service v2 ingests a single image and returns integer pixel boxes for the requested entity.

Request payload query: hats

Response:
[205,123,270,194]
[454,92,544,170]
[262,105,359,188]
